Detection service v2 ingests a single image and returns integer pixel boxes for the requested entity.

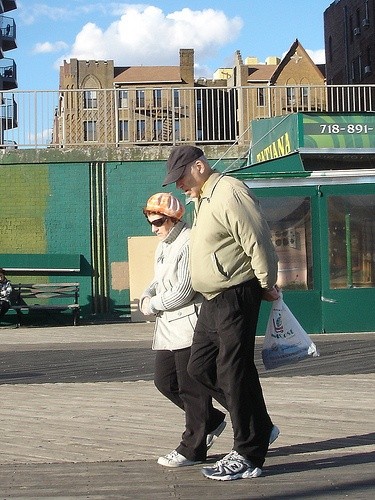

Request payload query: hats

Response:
[146,192,185,220]
[161,145,203,187]
[0,267,5,273]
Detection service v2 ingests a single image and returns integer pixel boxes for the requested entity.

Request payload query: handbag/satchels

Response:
[260,291,321,370]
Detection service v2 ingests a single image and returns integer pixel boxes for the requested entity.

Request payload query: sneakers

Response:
[157,450,203,468]
[269,426,280,446]
[201,450,262,483]
[205,420,226,453]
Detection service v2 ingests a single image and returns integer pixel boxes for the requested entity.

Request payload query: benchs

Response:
[10,281,80,328]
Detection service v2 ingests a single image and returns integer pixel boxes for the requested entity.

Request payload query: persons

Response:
[161,145,280,481]
[138,193,227,467]
[0,268,12,316]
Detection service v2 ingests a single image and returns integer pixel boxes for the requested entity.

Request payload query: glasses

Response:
[146,218,166,228]
